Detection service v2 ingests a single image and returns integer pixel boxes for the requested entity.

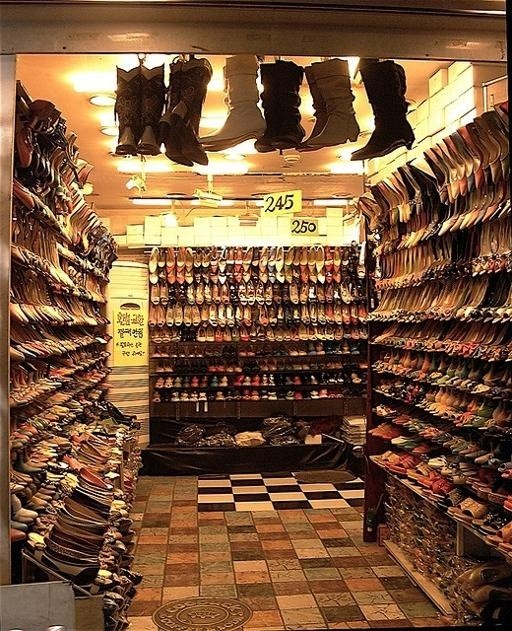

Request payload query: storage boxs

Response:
[363,61,508,189]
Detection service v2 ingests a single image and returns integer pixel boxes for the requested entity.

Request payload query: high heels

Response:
[150,281,369,345]
[148,247,364,285]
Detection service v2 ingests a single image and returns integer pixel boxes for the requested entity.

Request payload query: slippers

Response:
[368,452,511,566]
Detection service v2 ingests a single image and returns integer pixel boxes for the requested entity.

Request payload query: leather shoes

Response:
[475,586,512,603]
[468,566,511,585]
[151,340,368,403]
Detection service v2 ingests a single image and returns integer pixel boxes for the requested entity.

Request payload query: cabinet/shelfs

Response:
[362,456,511,621]
[146,391,369,448]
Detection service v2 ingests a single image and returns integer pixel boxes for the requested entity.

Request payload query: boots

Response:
[138,64,166,156]
[260,64,303,150]
[157,59,194,168]
[255,61,306,153]
[114,66,142,156]
[295,60,415,161]
[205,65,264,153]
[169,58,213,166]
[199,54,267,145]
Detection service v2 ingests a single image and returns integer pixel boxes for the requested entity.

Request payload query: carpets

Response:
[196,470,365,514]
